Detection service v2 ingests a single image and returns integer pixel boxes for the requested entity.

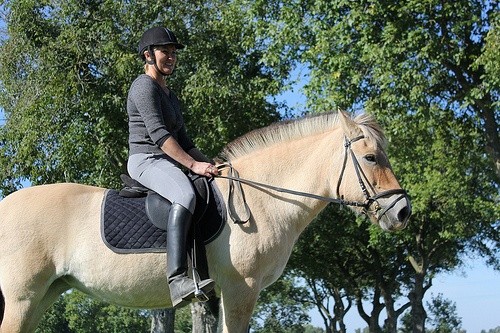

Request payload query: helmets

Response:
[139,26,185,61]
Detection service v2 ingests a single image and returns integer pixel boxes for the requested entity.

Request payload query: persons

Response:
[127,27,218,309]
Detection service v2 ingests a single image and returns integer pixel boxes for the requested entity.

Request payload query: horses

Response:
[0,104,412,333]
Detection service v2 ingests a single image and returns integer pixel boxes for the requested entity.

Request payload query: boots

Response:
[167,203,214,310]
